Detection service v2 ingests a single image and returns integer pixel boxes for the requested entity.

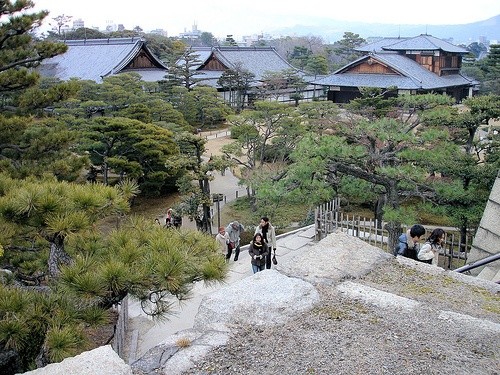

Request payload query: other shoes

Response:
[226,254,230,259]
[234,256,238,261]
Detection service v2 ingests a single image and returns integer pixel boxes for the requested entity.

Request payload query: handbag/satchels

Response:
[272,253,277,265]
[416,243,432,264]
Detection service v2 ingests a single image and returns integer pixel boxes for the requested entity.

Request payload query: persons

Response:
[226,221,241,261]
[253,217,276,269]
[248,233,270,274]
[215,227,230,259]
[394,225,426,256]
[417,228,446,265]
[154,208,183,231]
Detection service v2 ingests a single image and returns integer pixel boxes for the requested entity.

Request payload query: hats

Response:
[233,221,239,227]
[219,227,225,232]
